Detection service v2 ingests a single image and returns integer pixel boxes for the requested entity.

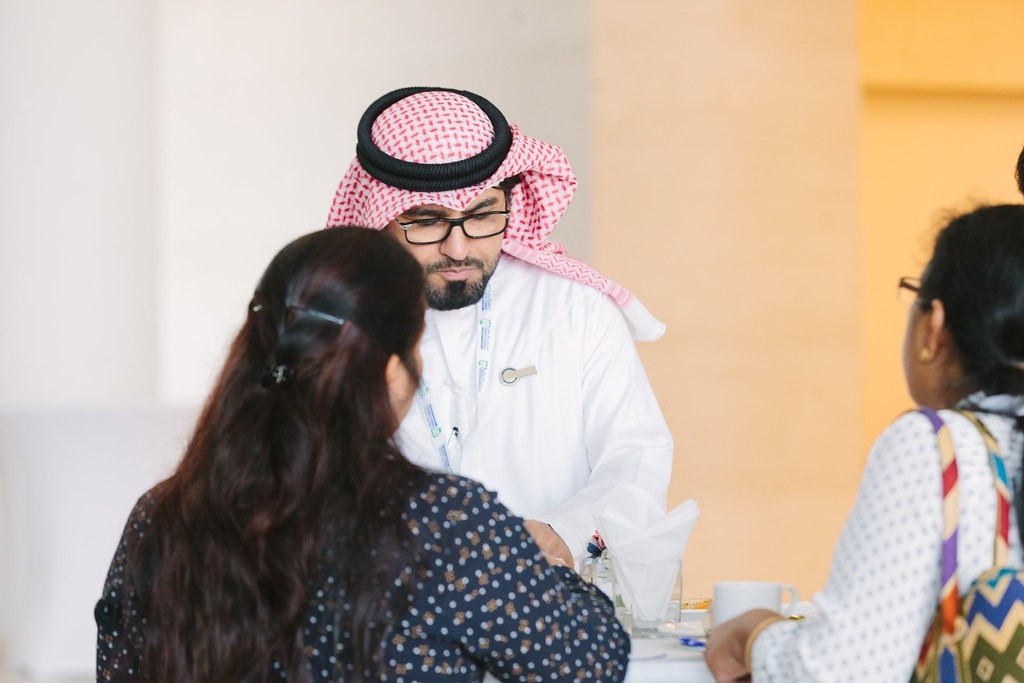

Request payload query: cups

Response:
[625,561,684,641]
[708,580,799,636]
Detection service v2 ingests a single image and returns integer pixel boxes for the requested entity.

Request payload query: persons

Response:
[324,86,674,601]
[95,225,632,683]
[704,204,1024,683]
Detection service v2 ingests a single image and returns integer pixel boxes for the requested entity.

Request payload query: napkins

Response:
[588,480,701,630]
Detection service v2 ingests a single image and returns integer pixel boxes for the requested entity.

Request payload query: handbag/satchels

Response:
[898,406,1024,683]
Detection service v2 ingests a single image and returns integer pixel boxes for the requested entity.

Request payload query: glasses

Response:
[394,195,511,245]
[897,277,963,326]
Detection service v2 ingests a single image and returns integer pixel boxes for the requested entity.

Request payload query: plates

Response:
[659,622,720,651]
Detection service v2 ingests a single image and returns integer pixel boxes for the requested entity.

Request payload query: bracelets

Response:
[745,614,806,676]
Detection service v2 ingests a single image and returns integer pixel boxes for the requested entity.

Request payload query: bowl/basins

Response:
[669,596,715,623]
